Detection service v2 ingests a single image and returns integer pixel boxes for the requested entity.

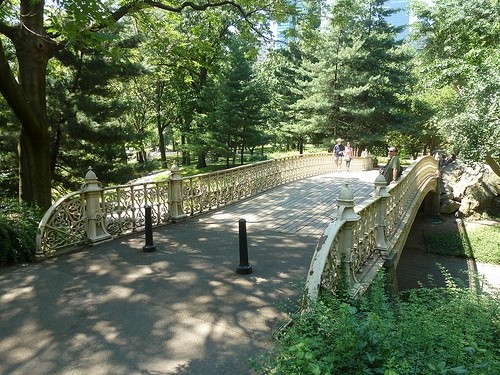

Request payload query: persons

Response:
[379,146,401,188]
[343,141,354,173]
[334,138,346,173]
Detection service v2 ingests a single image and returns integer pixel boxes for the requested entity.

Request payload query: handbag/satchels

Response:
[343,156,351,161]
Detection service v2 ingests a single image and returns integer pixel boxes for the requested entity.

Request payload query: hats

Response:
[335,138,344,143]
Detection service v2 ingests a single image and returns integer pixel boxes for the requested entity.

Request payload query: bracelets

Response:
[393,178,398,181]
